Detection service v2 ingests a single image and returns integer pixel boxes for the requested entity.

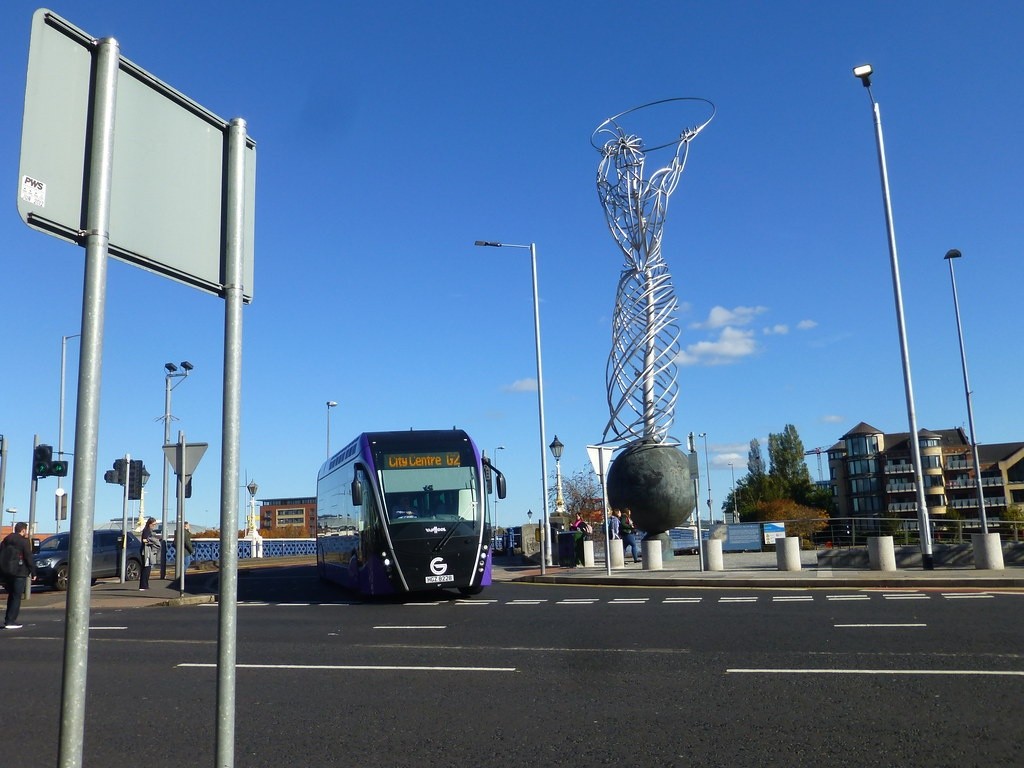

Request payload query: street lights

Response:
[244,477,264,540]
[473,239,554,571]
[6,508,18,533]
[854,62,937,571]
[698,432,713,525]
[548,434,569,533]
[728,462,740,524]
[944,247,1005,570]
[159,359,194,580]
[327,400,338,459]
[492,445,505,551]
[526,508,534,524]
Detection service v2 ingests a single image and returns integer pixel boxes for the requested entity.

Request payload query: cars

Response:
[31,529,143,591]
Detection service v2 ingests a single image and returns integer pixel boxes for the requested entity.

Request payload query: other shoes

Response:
[634,559,642,563]
[5,622,23,629]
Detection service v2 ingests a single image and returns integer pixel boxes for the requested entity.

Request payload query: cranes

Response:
[804,445,833,481]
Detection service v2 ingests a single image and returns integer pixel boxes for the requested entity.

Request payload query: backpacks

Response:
[602,518,613,534]
[0,536,24,575]
[578,520,592,535]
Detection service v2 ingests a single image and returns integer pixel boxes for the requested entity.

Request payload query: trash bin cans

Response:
[557,532,583,568]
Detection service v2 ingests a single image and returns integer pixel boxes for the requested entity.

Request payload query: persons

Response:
[174,522,193,579]
[392,496,418,519]
[0,522,37,629]
[569,513,587,567]
[139,518,156,591]
[608,510,629,565]
[619,508,642,563]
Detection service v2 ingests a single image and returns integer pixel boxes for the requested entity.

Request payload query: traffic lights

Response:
[32,444,68,476]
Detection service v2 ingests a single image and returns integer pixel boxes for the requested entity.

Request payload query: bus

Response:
[316,429,507,600]
